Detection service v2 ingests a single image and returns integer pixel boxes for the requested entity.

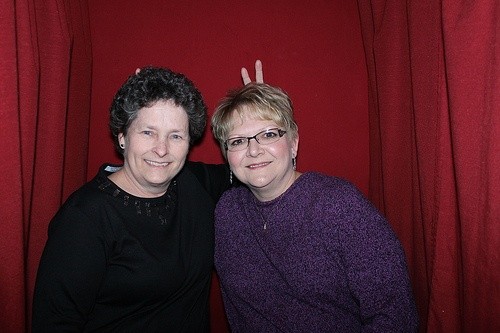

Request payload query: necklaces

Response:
[253,193,284,230]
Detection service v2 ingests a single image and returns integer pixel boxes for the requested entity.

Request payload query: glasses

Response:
[224,128,286,152]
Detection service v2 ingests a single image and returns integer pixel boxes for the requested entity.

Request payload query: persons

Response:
[134,68,419,333]
[32,61,264,333]
